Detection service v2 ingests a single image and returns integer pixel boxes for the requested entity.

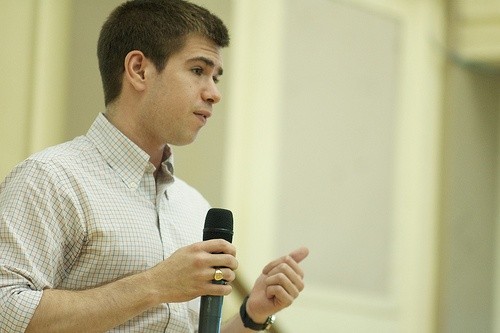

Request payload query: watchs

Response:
[239,296,275,330]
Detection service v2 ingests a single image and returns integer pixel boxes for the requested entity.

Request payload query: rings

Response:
[214,268,223,281]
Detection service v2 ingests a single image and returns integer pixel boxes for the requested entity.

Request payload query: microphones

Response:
[198,207,234,333]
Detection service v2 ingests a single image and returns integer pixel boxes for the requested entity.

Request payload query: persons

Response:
[0,0,309,333]
